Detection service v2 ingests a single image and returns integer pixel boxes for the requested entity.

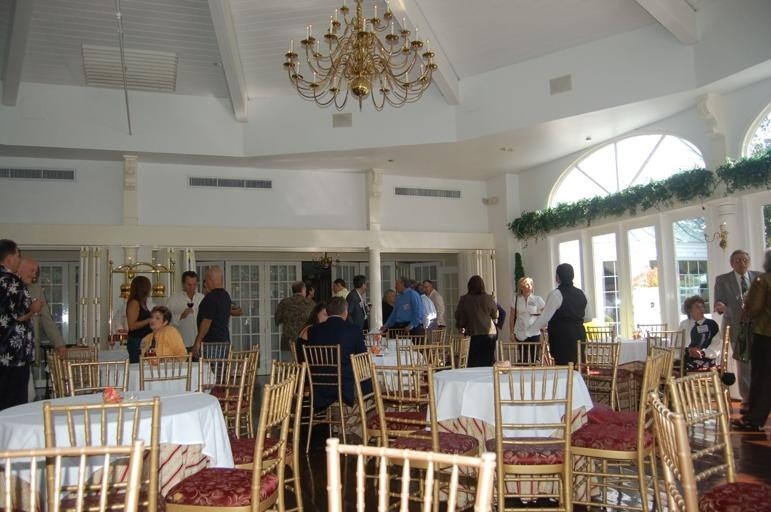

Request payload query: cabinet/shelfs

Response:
[109,260,176,346]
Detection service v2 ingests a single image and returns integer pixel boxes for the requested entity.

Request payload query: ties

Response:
[741,276,748,293]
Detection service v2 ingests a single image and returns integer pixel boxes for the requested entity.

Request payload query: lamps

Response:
[704,225,729,249]
[283,0,438,110]
[312,252,332,272]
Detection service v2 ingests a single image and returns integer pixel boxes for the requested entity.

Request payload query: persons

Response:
[714,249,771,431]
[16,257,66,403]
[509,277,546,365]
[527,263,592,365]
[275,276,371,351]
[124,264,243,385]
[380,275,446,354]
[455,275,506,367]
[0,238,44,410]
[307,296,372,412]
[673,294,723,375]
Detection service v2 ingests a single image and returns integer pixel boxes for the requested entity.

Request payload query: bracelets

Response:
[511,334,514,335]
[685,346,689,356]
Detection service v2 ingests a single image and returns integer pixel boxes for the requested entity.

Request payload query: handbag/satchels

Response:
[732,321,753,362]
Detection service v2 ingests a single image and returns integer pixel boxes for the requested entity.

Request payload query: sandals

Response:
[731,416,759,429]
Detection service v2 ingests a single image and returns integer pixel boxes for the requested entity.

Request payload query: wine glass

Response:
[187,299,194,308]
[368,303,373,310]
[146,348,155,357]
